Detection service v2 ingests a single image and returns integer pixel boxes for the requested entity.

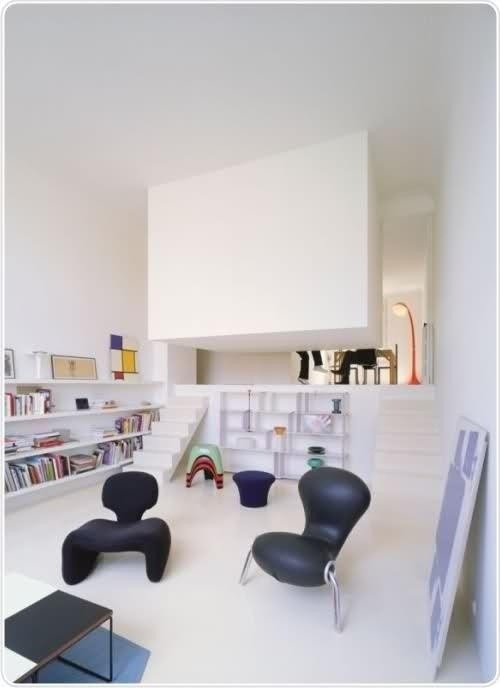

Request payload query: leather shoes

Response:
[331,370,345,376]
[335,379,349,384]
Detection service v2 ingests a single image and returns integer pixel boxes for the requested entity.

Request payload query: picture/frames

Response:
[51,355,97,380]
[4,348,15,379]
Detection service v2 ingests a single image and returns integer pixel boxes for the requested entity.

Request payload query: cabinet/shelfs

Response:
[220,392,347,480]
[3,380,166,498]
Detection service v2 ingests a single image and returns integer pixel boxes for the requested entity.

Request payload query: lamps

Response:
[393,302,421,385]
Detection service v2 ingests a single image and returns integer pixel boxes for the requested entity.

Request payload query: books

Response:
[4,388,160,494]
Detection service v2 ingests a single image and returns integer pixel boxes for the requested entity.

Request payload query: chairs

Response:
[240,468,371,633]
[61,472,171,584]
[333,343,398,384]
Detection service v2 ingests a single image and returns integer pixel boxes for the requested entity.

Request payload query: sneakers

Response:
[298,377,309,384]
[314,366,328,373]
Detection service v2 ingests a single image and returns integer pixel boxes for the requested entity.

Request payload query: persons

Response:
[331,349,378,384]
[296,351,329,385]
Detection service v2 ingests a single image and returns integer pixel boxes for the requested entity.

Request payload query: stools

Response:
[187,444,224,489]
[233,471,276,507]
